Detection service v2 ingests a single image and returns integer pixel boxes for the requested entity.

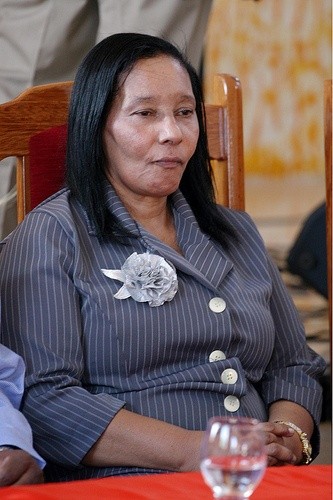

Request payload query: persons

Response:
[0,343,46,486]
[0,32,327,483]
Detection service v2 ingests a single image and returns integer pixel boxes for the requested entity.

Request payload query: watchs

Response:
[270,419,314,465]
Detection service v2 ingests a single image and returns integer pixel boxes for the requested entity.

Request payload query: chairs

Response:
[1,73,245,226]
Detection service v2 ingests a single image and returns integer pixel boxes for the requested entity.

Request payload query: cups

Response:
[198,417,268,500]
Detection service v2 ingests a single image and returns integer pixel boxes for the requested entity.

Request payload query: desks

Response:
[0,465,333,500]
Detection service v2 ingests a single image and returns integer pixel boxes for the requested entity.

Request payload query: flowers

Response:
[101,251,178,308]
